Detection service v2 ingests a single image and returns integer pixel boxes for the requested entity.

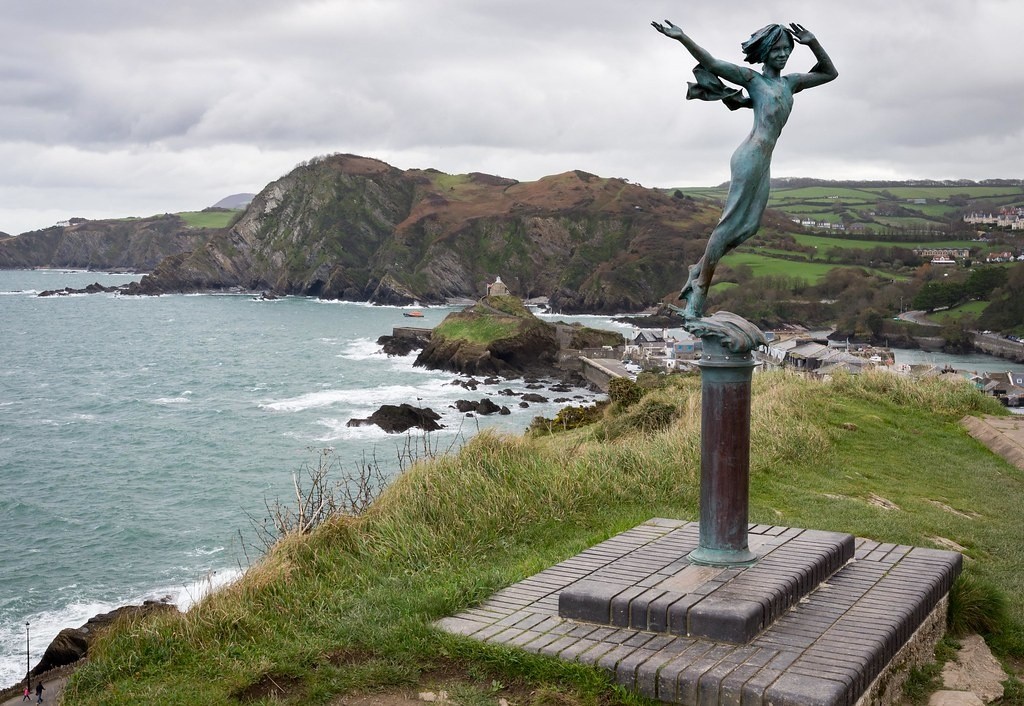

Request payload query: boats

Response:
[402,312,424,317]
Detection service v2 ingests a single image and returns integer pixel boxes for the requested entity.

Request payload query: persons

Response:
[651,19,839,354]
